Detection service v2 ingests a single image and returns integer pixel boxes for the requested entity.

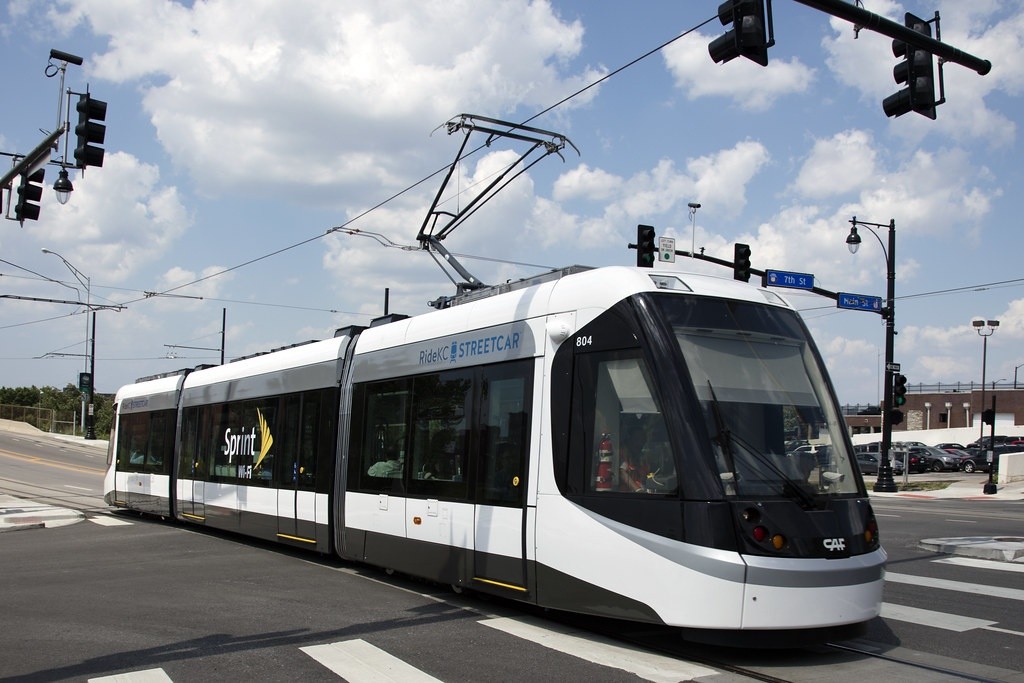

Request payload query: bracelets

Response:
[635,488,642,492]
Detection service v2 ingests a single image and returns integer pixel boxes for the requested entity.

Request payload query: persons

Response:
[367,445,403,479]
[492,450,520,500]
[618,403,678,493]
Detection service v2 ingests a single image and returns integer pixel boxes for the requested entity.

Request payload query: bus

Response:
[101,266,888,630]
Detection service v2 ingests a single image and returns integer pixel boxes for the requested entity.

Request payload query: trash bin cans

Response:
[819,464,837,485]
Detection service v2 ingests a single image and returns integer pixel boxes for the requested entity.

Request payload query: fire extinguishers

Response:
[597,432,614,488]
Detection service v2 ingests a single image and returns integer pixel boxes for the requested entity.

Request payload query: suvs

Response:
[784,440,824,457]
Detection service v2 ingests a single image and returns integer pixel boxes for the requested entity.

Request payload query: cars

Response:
[959,445,1024,474]
[854,441,927,453]
[854,453,903,476]
[892,451,926,474]
[909,447,960,472]
[935,435,1024,455]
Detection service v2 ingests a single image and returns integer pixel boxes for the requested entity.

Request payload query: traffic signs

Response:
[885,362,900,373]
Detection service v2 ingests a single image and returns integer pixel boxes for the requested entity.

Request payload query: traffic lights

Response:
[15,168,45,228]
[75,91,108,169]
[895,373,907,405]
[734,243,751,283]
[708,0,769,68]
[891,409,903,424]
[637,225,656,268]
[882,12,937,121]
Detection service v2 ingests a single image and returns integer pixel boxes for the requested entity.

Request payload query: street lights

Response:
[973,320,999,455]
[846,216,897,492]
[41,248,91,433]
